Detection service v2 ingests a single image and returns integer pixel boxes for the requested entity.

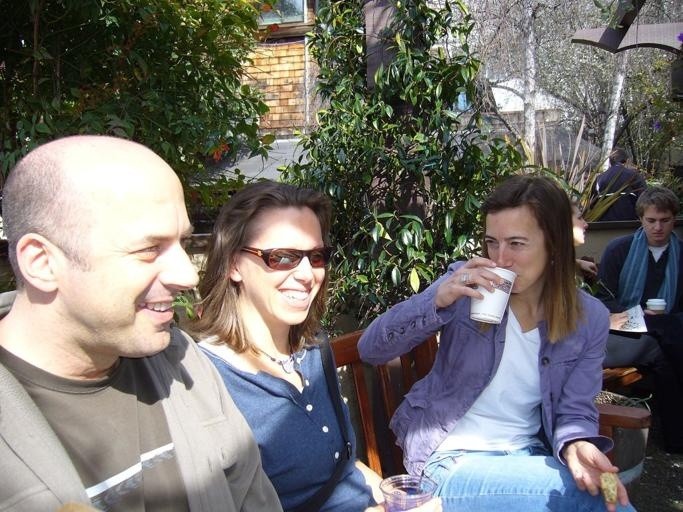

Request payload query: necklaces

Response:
[255,343,296,374]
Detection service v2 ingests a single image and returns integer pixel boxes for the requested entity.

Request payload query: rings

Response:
[460,272,471,285]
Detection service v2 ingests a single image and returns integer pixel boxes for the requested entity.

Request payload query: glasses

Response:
[239,246,336,270]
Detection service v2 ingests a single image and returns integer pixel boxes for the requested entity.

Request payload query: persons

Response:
[0,135,284,512]
[563,199,682,452]
[196,180,443,511]
[597,187,683,325]
[589,150,647,222]
[358,173,639,511]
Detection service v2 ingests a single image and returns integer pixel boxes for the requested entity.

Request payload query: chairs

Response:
[599,360,644,390]
[329,330,652,479]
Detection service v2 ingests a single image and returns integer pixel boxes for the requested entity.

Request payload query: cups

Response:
[645,299,668,314]
[470,264,518,326]
[378,473,439,512]
